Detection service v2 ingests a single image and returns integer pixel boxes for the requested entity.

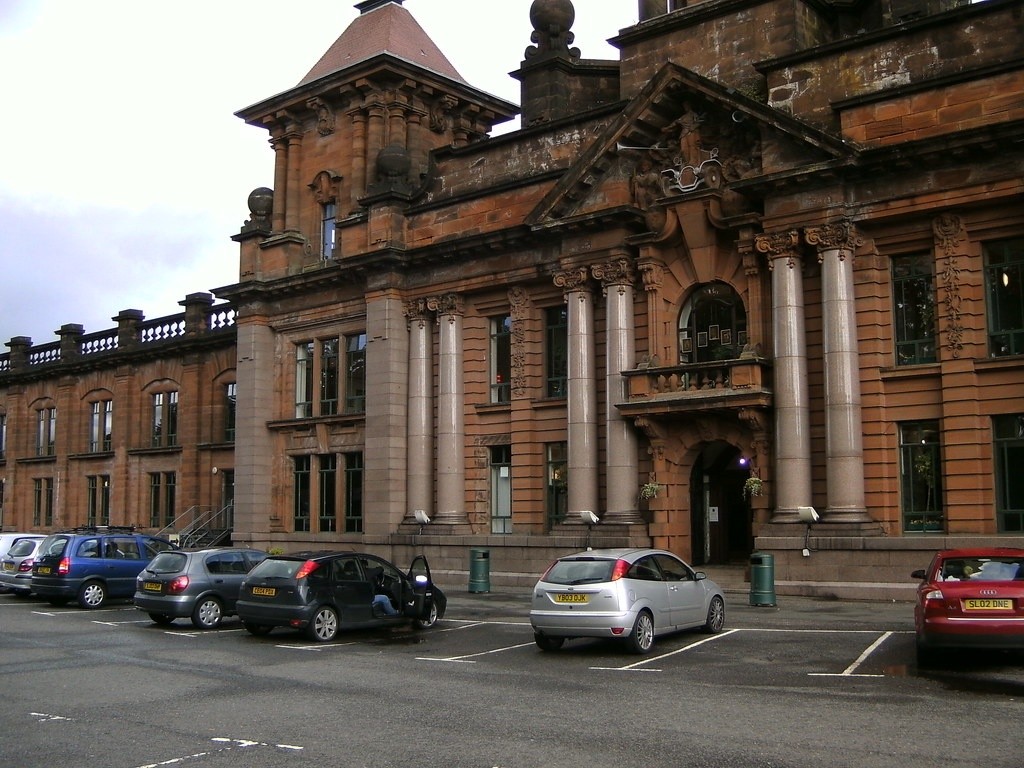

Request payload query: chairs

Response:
[635,562,656,579]
[943,560,973,580]
[343,561,358,579]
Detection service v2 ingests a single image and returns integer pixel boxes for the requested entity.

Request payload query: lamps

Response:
[579,509,600,551]
[414,510,430,534]
[798,507,821,556]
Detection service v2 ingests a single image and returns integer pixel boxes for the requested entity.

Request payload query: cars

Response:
[236,551,447,641]
[134,547,270,631]
[30,532,181,609]
[911,547,1024,667]
[530,547,728,655]
[0,534,126,596]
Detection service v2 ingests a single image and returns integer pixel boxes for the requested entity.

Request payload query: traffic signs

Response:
[168,534,180,547]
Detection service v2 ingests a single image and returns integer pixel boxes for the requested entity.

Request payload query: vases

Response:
[899,357,935,364]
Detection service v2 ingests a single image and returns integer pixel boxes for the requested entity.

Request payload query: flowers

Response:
[893,280,936,362]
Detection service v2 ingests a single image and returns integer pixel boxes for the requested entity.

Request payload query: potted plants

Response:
[742,478,764,500]
[634,482,664,507]
[908,446,940,531]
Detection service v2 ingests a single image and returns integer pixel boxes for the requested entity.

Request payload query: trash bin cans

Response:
[468,548,490,594]
[750,553,776,606]
